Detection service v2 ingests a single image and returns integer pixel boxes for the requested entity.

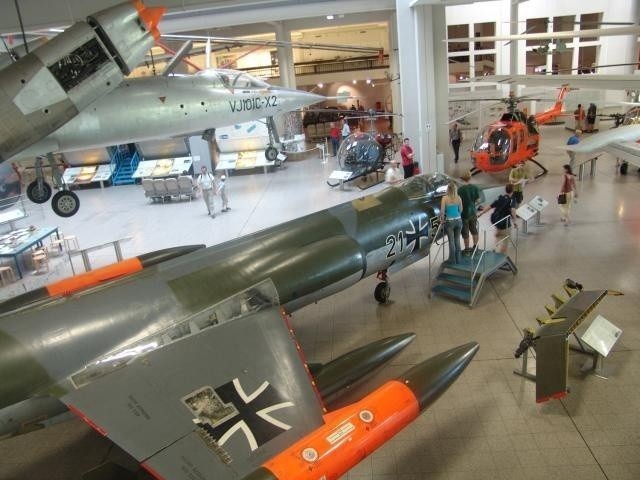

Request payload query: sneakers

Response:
[462,245,478,257]
[208,207,232,219]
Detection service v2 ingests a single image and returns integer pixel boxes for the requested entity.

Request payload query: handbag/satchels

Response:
[558,195,567,204]
[491,195,515,227]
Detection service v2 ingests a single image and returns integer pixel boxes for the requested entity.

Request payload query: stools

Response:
[0,234,80,286]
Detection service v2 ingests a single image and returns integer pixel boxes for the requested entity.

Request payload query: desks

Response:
[0,209,62,279]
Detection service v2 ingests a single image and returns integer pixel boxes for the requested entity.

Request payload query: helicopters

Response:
[442,63,640,192]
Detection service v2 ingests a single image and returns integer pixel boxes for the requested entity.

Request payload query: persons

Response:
[567,130,582,164]
[478,183,517,254]
[556,165,577,227]
[439,171,481,265]
[329,119,351,157]
[197,166,231,218]
[401,138,420,179]
[574,102,596,133]
[509,161,528,208]
[449,123,463,164]
[384,159,401,184]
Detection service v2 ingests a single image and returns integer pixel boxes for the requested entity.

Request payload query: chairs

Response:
[142,176,199,204]
